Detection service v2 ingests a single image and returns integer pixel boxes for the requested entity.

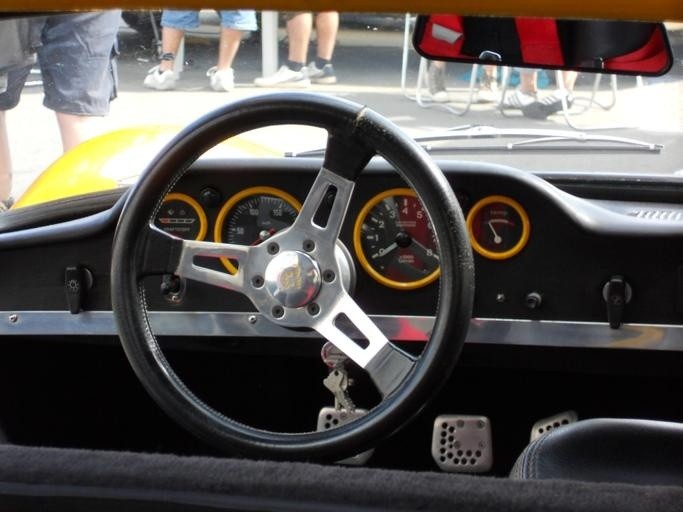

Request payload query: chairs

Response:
[508,419,682,486]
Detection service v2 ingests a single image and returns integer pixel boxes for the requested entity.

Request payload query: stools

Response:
[403,12,648,130]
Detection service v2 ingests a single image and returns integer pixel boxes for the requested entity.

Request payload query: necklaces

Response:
[0,9,123,216]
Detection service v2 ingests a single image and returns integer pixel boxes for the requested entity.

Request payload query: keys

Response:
[322,353,356,413]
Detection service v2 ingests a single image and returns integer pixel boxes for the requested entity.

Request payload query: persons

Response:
[423,59,498,104]
[498,66,579,121]
[140,10,258,95]
[252,11,341,90]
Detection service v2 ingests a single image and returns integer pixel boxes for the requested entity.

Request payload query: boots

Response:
[402,63,451,103]
[474,66,499,102]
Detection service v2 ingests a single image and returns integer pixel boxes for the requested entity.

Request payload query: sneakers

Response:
[207,66,236,91]
[309,61,337,86]
[494,85,573,117]
[143,66,179,90]
[254,64,313,89]
